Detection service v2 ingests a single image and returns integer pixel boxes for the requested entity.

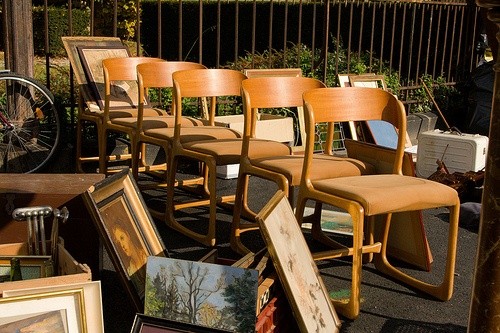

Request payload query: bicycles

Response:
[0,69,64,174]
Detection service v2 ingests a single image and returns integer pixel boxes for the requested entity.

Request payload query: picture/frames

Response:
[255,190,342,333]
[81,170,171,314]
[0,255,88,333]
[130,314,237,333]
[338,73,379,143]
[349,75,388,143]
[76,45,151,111]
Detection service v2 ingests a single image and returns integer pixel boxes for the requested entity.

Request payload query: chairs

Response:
[75,57,461,319]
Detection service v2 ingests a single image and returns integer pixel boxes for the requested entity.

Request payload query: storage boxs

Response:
[0,241,92,293]
[417,130,489,184]
[191,246,275,333]
[0,173,109,279]
[196,114,295,178]
